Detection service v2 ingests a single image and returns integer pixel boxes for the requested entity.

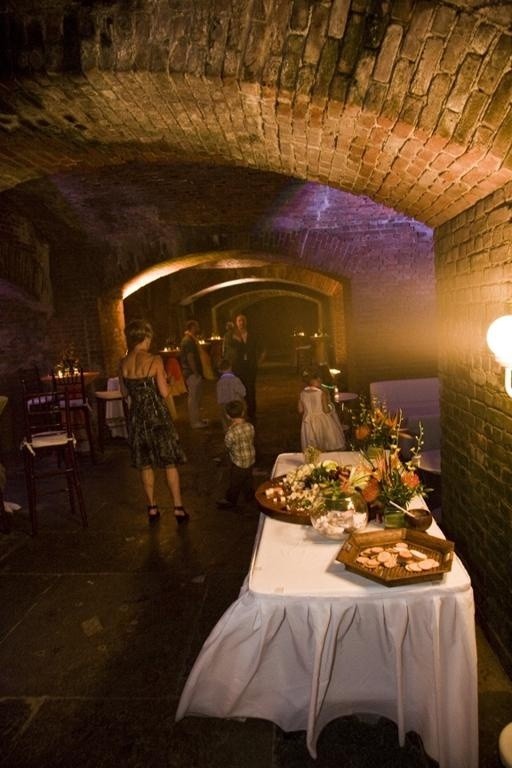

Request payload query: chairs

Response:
[20,366,133,536]
[295,331,313,375]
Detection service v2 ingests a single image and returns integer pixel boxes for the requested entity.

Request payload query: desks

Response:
[159,335,225,397]
[412,449,442,474]
[308,334,331,370]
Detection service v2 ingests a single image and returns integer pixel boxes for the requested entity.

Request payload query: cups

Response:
[384,507,407,528]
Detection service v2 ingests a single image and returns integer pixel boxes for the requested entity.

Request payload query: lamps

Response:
[486,315,512,399]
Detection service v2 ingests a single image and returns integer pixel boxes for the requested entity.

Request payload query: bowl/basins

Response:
[404,508,431,531]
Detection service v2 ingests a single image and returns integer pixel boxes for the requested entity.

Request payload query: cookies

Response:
[356,543,440,572]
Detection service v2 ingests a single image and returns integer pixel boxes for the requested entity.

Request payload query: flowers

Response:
[333,391,434,508]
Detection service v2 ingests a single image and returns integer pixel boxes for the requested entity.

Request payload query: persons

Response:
[118,320,190,525]
[222,322,234,356]
[298,366,346,451]
[179,319,209,429]
[227,313,263,421]
[318,365,337,410]
[216,400,256,508]
[217,359,246,434]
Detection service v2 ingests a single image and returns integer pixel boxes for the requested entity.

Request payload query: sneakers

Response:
[215,497,234,505]
[190,420,209,430]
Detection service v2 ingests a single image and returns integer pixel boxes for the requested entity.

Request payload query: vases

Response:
[383,499,409,530]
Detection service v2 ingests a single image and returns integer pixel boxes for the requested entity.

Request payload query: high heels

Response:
[148,505,161,523]
[173,506,191,524]
